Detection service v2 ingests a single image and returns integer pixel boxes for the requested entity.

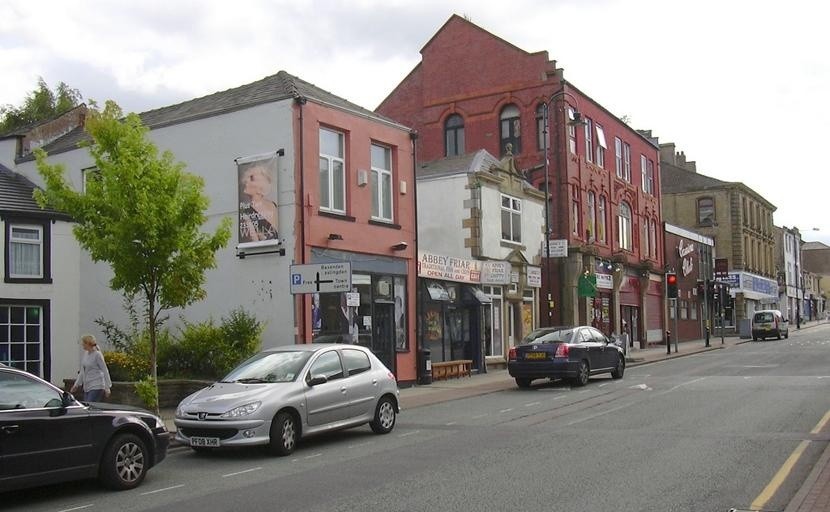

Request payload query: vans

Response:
[751,309,789,341]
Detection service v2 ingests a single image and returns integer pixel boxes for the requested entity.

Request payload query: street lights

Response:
[543,92,588,324]
[793,227,820,329]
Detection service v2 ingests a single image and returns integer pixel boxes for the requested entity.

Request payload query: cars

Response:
[173,343,401,457]
[508,325,625,389]
[0,364,170,506]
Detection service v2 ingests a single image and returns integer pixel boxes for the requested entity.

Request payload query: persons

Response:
[70,334,112,402]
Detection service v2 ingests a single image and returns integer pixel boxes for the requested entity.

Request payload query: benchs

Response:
[432,360,473,381]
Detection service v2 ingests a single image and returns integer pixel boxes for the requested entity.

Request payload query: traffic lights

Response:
[665,273,678,299]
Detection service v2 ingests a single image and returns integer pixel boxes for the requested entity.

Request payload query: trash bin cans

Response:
[740,319,752,339]
[417,347,432,384]
